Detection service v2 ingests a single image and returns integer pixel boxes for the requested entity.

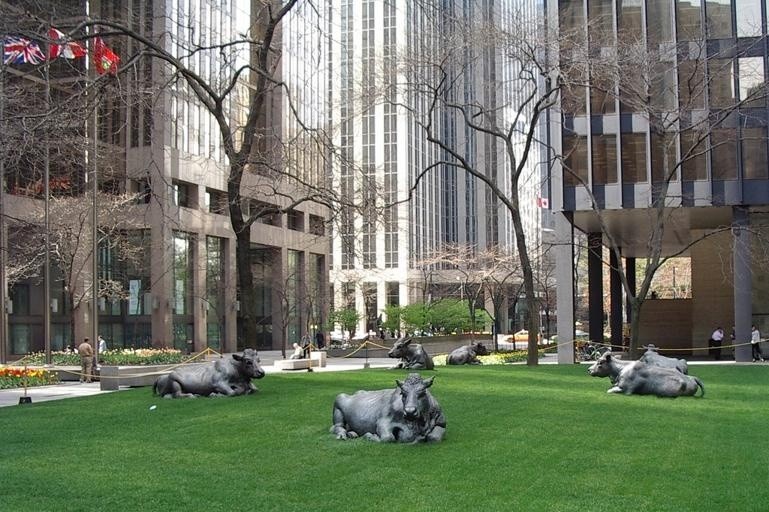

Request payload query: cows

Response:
[446,341,491,365]
[638,344,689,376]
[586,350,705,397]
[152,348,265,399]
[387,337,435,370]
[329,372,446,444]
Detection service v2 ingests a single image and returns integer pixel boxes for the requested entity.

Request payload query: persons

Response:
[312,329,329,352]
[96,334,108,353]
[79,337,94,384]
[300,332,312,360]
[710,321,766,362]
[288,342,303,360]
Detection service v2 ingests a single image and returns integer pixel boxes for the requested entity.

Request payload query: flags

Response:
[0,33,45,68]
[48,26,84,60]
[89,31,122,74]
